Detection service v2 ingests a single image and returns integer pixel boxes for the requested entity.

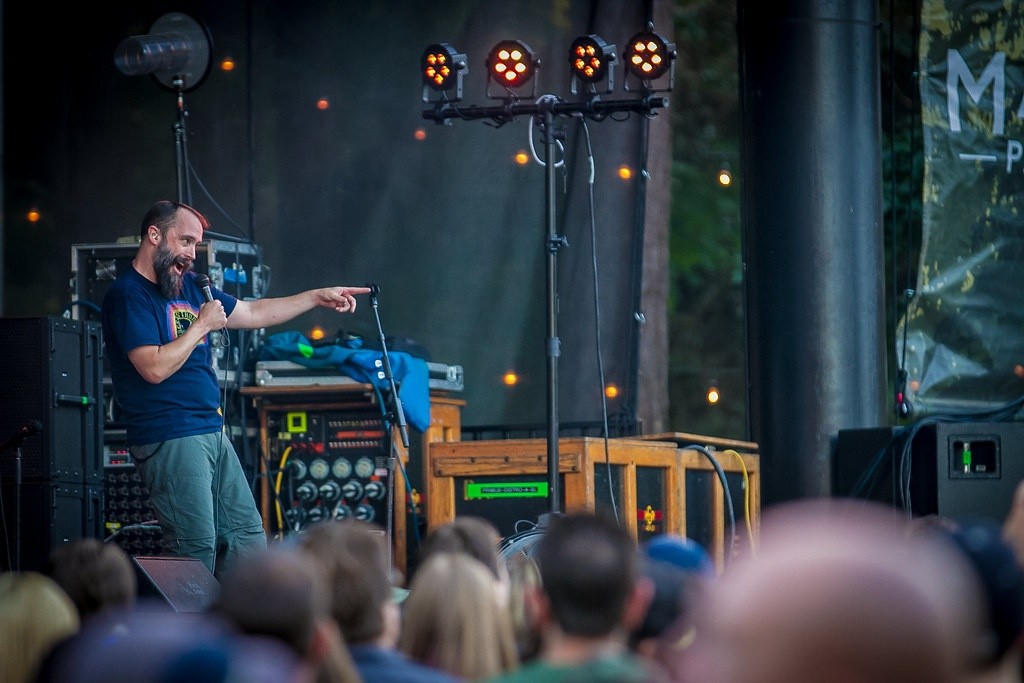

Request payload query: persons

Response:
[102,199,372,598]
[0,476,1024,683]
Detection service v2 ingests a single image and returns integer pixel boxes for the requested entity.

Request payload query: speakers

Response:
[0,313,104,485]
[132,556,223,614]
[0,485,105,615]
[838,420,1024,528]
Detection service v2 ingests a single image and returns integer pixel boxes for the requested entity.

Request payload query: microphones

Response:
[196,274,224,336]
[4,421,43,449]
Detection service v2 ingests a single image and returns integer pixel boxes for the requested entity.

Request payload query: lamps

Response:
[485,40,540,101]
[569,33,619,97]
[422,41,468,105]
[622,22,679,94]
